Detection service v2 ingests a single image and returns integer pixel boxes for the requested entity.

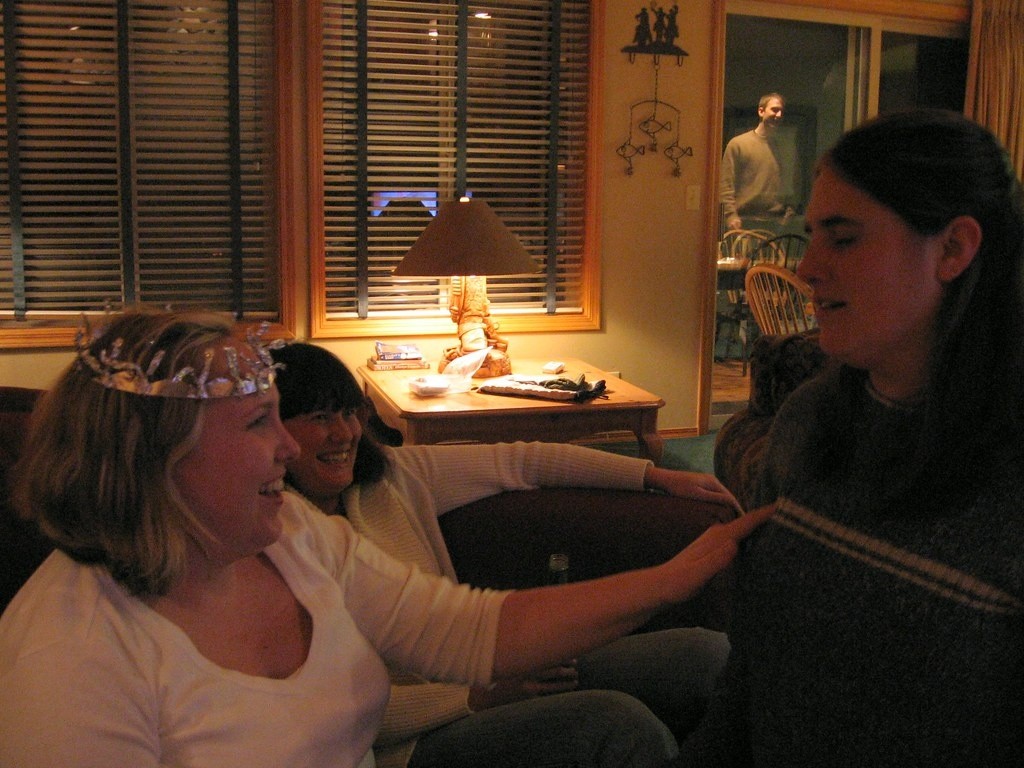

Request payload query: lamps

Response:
[390,198,544,379]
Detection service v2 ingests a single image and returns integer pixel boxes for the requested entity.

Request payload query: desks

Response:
[717,256,802,291]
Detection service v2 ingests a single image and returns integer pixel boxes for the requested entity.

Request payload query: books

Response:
[366,339,431,371]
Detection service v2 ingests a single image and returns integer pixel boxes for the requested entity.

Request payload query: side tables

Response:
[355,358,667,468]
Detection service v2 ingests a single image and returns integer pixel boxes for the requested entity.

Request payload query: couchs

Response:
[0,412,733,768]
[713,329,826,504]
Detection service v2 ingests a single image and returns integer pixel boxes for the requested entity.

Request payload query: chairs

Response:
[744,263,816,335]
[715,232,809,378]
[718,229,785,305]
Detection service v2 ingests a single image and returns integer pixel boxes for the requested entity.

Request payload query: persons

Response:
[0,311,776,768]
[719,94,795,360]
[730,109,1024,766]
[268,343,737,768]
